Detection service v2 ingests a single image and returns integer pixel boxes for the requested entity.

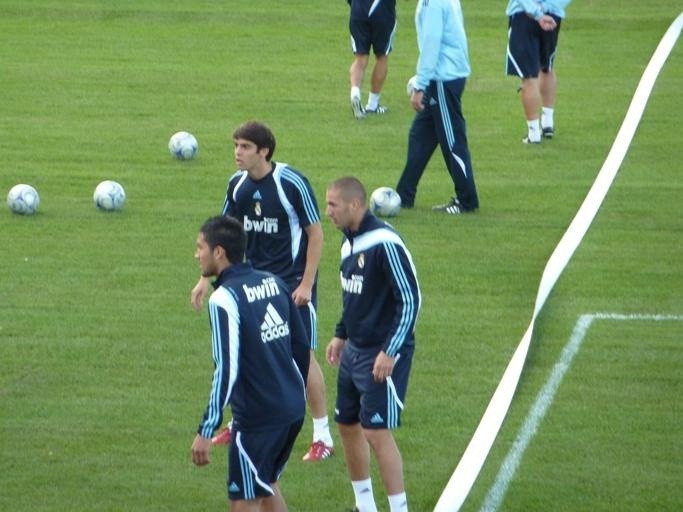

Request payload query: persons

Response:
[191,121,337,460]
[325,177,421,511]
[191,215,307,512]
[396,0,479,215]
[506,0,572,144]
[346,1,398,120]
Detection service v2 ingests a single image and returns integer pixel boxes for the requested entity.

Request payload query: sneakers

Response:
[212,423,230,443]
[366,104,387,114]
[431,198,475,214]
[350,97,364,119]
[522,128,552,143]
[302,440,334,461]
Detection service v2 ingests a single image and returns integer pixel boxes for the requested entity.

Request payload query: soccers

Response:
[7,183,39,216]
[168,131,198,159]
[407,74,418,97]
[369,186,401,217]
[93,180,127,212]
